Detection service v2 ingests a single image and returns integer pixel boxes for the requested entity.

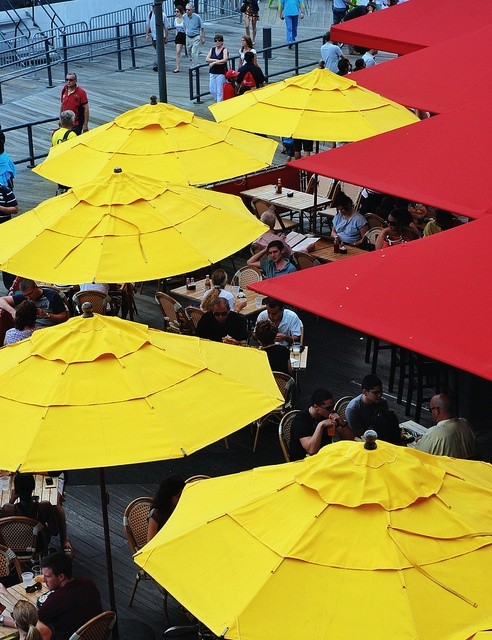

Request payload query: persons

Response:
[290,389,355,462]
[221,70,240,101]
[146,478,183,543]
[252,211,292,255]
[331,196,369,250]
[205,35,228,103]
[52,110,78,196]
[339,0,376,55]
[280,0,304,49]
[196,298,247,347]
[422,211,456,238]
[1,473,58,556]
[293,139,313,160]
[414,393,477,458]
[353,59,366,72]
[243,0,259,45]
[3,301,42,346]
[1,279,69,330]
[47,471,73,552]
[335,59,349,76]
[236,51,265,95]
[252,299,302,344]
[199,268,247,314]
[320,32,352,74]
[0,184,19,224]
[173,5,186,73]
[375,209,419,250]
[345,375,388,439]
[283,138,294,162]
[246,240,297,278]
[0,133,17,191]
[0,553,103,640]
[408,108,420,117]
[0,600,52,640]
[255,320,293,376]
[182,3,205,76]
[332,0,355,45]
[145,3,169,72]
[60,72,89,136]
[407,201,427,236]
[362,48,378,67]
[238,35,257,68]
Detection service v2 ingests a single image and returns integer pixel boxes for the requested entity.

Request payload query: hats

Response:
[225,70,241,78]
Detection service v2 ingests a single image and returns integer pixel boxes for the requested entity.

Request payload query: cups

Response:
[255,297,263,309]
[231,286,240,298]
[22,572,33,588]
[327,419,336,436]
[292,342,301,356]
[32,565,44,583]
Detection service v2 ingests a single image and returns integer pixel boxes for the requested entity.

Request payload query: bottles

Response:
[334,235,339,253]
[237,289,247,309]
[190,277,195,288]
[204,275,211,292]
[278,178,282,193]
[186,278,189,288]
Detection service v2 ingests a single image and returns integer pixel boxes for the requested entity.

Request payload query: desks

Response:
[311,246,368,262]
[306,239,334,257]
[242,185,294,211]
[0,575,56,639]
[0,471,60,508]
[170,277,269,315]
[272,190,331,231]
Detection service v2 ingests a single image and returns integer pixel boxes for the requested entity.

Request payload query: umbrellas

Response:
[30,94,280,187]
[1,167,270,321]
[0,301,286,640]
[132,430,492,640]
[207,59,421,234]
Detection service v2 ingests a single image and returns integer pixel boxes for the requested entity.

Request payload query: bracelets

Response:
[44,312,51,319]
[353,242,355,247]
[234,310,239,313]
[341,420,348,427]
[284,335,290,342]
[301,11,304,13]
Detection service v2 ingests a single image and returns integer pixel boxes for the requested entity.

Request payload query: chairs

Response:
[69,610,117,640]
[232,265,261,287]
[183,305,205,328]
[248,196,299,233]
[0,543,22,579]
[332,396,354,416]
[360,227,383,250]
[123,497,168,624]
[317,180,365,237]
[291,252,321,269]
[290,173,335,232]
[183,473,212,481]
[248,371,295,452]
[0,517,48,573]
[278,408,321,461]
[364,212,388,228]
[154,291,185,327]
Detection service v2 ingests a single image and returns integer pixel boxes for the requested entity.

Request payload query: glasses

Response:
[66,78,75,82]
[185,9,191,11]
[211,312,228,317]
[175,10,179,13]
[319,405,334,411]
[215,39,222,43]
[428,407,439,413]
[388,219,398,227]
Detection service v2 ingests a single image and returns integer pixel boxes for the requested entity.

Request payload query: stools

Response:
[386,347,431,404]
[364,332,395,375]
[404,353,440,421]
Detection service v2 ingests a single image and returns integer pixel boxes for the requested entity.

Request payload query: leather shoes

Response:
[62,539,73,559]
